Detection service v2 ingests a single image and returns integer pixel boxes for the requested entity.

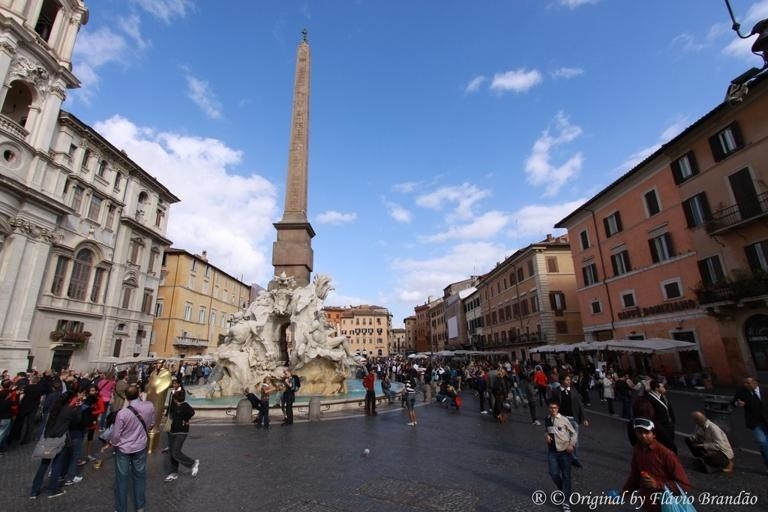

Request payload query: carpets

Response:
[752,390,759,400]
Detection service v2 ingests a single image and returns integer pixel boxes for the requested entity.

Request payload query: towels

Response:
[705,400,733,442]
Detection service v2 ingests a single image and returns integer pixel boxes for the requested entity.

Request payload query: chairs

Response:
[29,456,199,500]
[721,461,734,473]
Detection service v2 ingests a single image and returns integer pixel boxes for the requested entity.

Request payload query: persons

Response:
[1,359,210,499]
[254,374,277,430]
[215,288,367,382]
[541,400,580,512]
[278,369,297,427]
[733,373,768,476]
[619,415,692,512]
[685,406,736,476]
[103,385,157,512]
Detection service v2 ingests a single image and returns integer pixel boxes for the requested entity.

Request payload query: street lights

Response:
[31,438,64,459]
[660,481,696,512]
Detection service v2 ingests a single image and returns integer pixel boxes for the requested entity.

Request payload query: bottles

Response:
[632,417,653,431]
